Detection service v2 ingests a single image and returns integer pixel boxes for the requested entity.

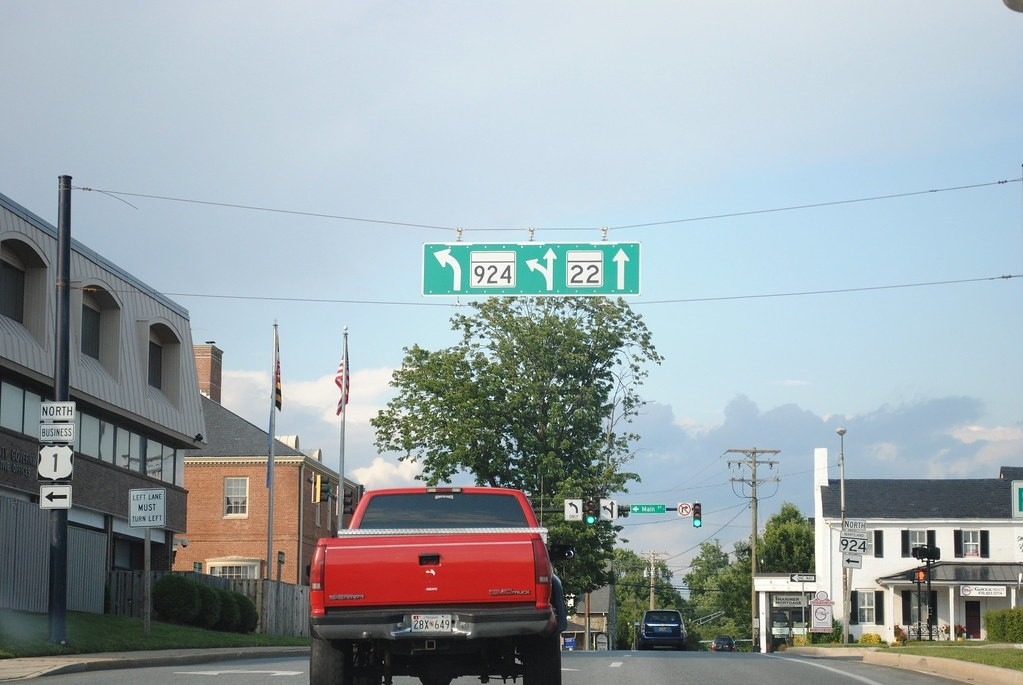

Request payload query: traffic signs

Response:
[419,241,641,298]
[629,503,666,514]
[790,573,817,583]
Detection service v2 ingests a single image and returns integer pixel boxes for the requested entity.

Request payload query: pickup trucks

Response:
[307,487,568,685]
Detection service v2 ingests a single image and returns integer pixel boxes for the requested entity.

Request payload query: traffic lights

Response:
[693,501,701,527]
[918,569,927,580]
[585,499,596,525]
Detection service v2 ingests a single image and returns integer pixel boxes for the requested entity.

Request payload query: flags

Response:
[335,352,349,416]
[271,345,281,411]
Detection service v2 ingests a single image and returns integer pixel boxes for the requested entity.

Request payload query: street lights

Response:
[836,426,848,619]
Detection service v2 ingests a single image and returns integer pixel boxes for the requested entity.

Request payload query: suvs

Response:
[710,635,736,652]
[638,609,687,651]
